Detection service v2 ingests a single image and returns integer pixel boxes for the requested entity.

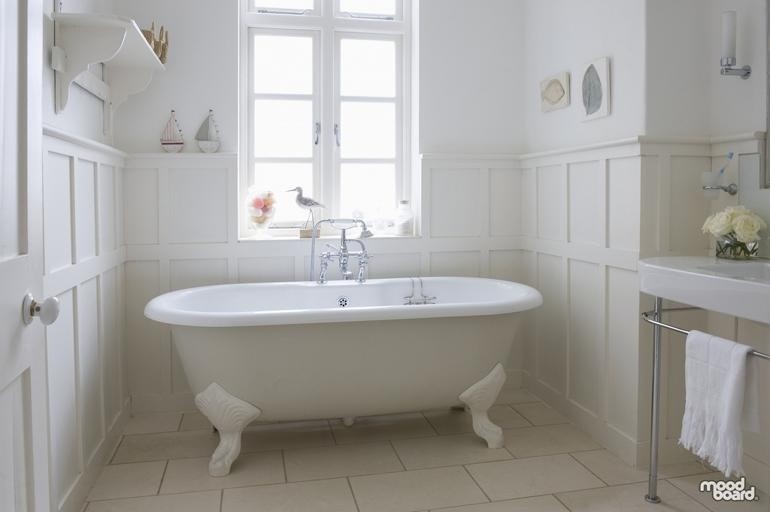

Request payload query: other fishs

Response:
[542,79,565,105]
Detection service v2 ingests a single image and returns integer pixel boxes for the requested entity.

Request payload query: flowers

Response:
[701,204,767,258]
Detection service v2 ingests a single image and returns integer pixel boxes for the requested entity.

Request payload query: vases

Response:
[714,240,759,258]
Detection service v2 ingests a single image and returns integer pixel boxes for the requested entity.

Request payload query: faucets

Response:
[317,252,334,284]
[354,250,370,284]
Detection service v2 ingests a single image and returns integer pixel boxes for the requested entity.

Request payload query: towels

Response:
[676,330,759,479]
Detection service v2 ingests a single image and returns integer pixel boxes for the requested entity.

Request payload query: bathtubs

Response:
[140,275,546,426]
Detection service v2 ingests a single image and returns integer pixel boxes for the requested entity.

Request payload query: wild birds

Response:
[286,187,326,230]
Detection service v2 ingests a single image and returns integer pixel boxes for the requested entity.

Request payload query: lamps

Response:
[719,10,751,79]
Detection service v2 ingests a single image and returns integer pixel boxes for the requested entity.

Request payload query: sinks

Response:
[696,261,770,285]
